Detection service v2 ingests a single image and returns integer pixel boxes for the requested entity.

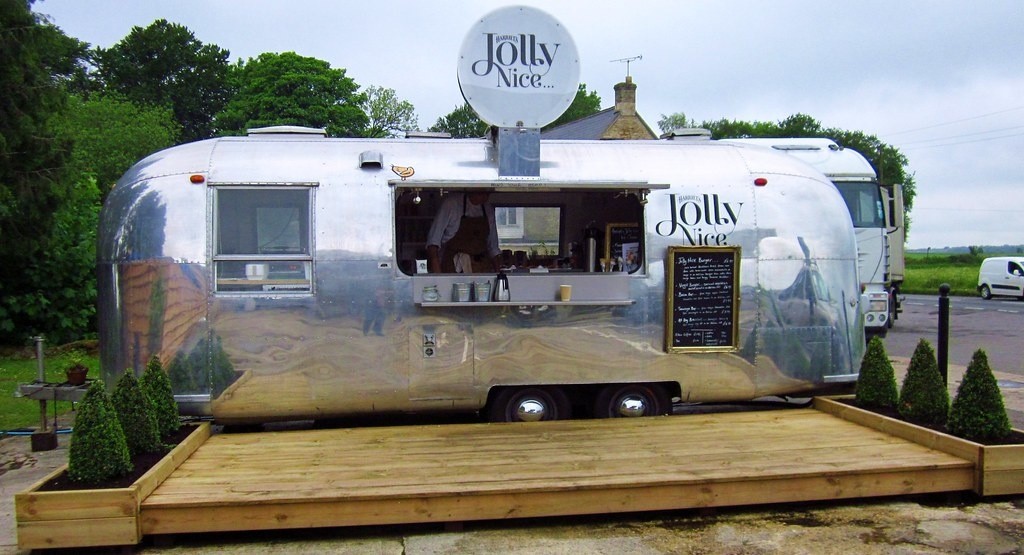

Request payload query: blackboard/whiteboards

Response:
[665,245,738,353]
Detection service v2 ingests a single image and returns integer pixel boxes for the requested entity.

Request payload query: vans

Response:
[975,255,1024,299]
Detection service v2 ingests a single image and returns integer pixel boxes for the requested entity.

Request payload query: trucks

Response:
[92,5,869,427]
[718,133,907,328]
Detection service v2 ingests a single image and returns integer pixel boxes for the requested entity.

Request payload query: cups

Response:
[472,282,491,302]
[560,285,572,302]
[453,282,473,303]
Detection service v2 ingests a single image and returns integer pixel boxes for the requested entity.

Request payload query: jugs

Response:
[581,227,600,273]
[493,269,511,302]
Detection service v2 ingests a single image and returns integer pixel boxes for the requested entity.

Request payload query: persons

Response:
[426,190,501,275]
[361,282,387,337]
